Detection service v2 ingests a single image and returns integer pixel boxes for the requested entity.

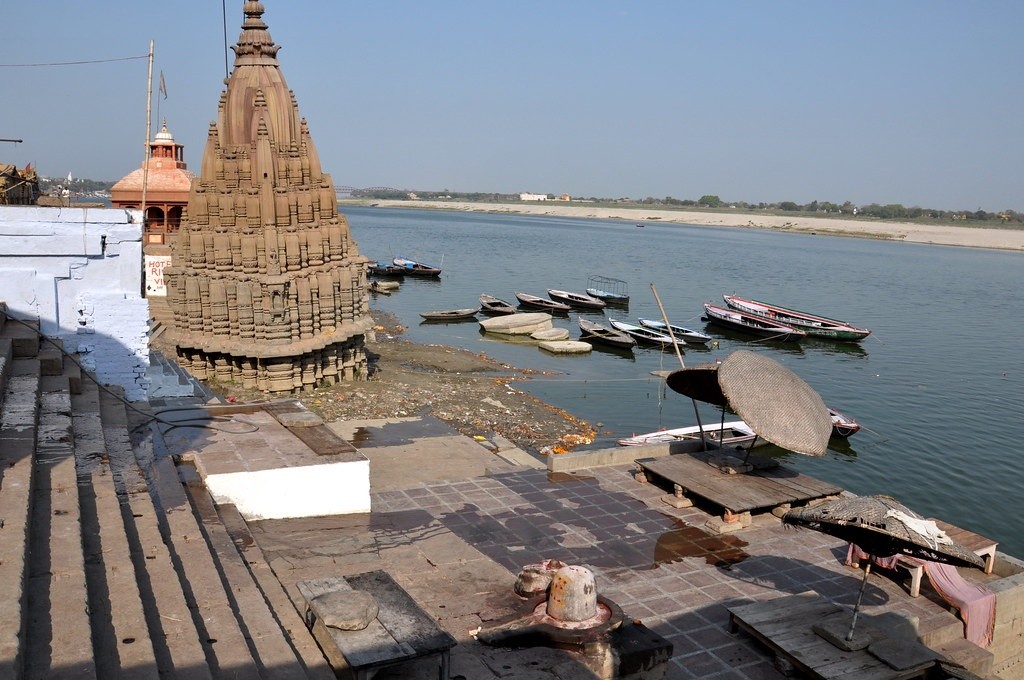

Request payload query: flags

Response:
[160,70,168,100]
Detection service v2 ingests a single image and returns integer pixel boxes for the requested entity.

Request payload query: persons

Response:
[57,187,70,199]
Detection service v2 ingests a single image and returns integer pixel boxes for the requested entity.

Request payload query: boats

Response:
[578,315,637,349]
[546,288,607,309]
[418,306,481,320]
[392,255,442,276]
[586,274,629,305]
[480,292,517,314]
[616,420,768,451]
[722,293,871,341]
[419,317,479,326]
[638,316,712,343]
[609,317,690,348]
[636,224,644,227]
[708,362,860,436]
[370,262,416,276]
[702,302,809,338]
[514,290,572,314]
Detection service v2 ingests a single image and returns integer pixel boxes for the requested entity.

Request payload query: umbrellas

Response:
[782,495,987,640]
[666,350,833,464]
